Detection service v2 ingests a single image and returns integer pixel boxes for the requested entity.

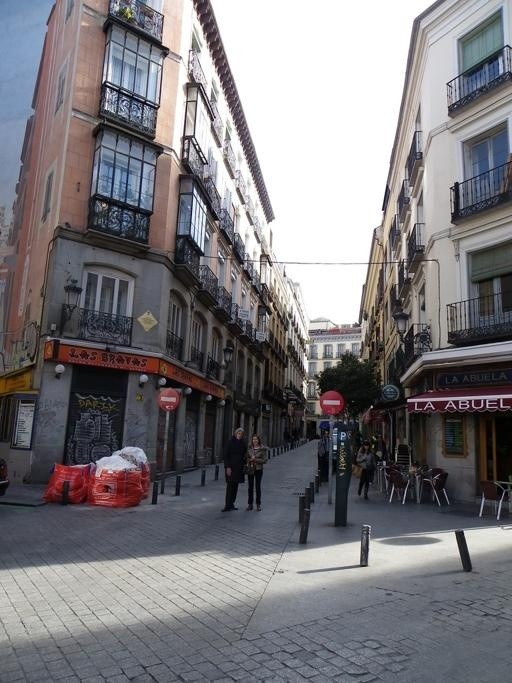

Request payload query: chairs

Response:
[479,481,512,521]
[385,465,451,507]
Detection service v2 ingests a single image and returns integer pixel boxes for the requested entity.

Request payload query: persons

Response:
[352,430,387,500]
[246,434,269,511]
[221,427,247,511]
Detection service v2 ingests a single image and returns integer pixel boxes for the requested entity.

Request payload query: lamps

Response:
[139,375,225,406]
[223,347,233,369]
[63,279,82,320]
[55,364,65,379]
[392,309,409,343]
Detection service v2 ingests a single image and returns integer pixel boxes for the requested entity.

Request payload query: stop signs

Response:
[157,387,180,412]
[318,390,345,416]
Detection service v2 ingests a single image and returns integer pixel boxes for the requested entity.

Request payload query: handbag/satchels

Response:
[243,460,255,475]
[350,460,363,477]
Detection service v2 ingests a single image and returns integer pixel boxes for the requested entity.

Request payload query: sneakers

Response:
[246,505,254,511]
[221,504,238,512]
[356,487,369,500]
[256,505,261,511]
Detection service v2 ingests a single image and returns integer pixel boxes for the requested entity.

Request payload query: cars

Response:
[0,456,10,496]
[330,426,338,468]
